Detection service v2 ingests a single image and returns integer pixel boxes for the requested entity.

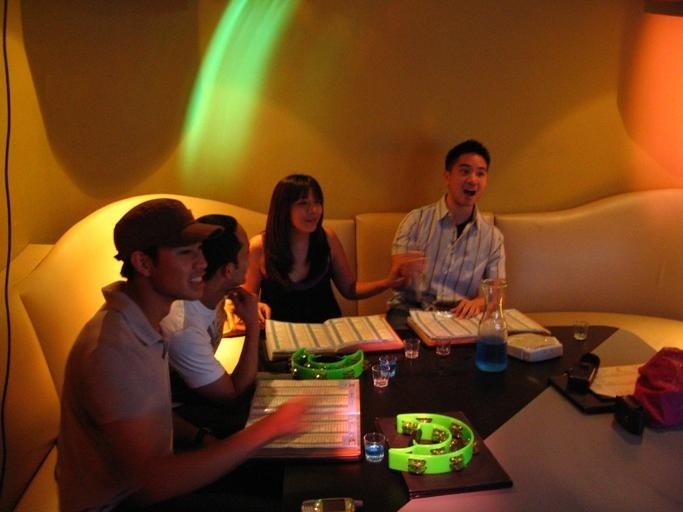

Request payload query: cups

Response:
[364,433,386,463]
[371,355,397,387]
[573,319,588,340]
[436,336,450,356]
[404,338,420,359]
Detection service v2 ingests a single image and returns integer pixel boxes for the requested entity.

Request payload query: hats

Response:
[113,197,224,251]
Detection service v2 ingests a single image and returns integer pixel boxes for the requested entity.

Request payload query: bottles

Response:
[475,278,508,372]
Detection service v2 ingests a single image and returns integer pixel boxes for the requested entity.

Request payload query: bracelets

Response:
[197,427,212,441]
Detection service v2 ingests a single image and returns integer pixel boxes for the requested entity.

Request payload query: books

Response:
[406,308,551,347]
[245,378,362,460]
[264,314,404,362]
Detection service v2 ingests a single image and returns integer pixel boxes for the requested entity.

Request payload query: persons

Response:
[159,214,260,441]
[58,197,309,512]
[240,175,419,339]
[386,139,506,330]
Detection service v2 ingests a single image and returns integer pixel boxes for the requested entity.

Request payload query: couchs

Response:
[0,188,683,512]
